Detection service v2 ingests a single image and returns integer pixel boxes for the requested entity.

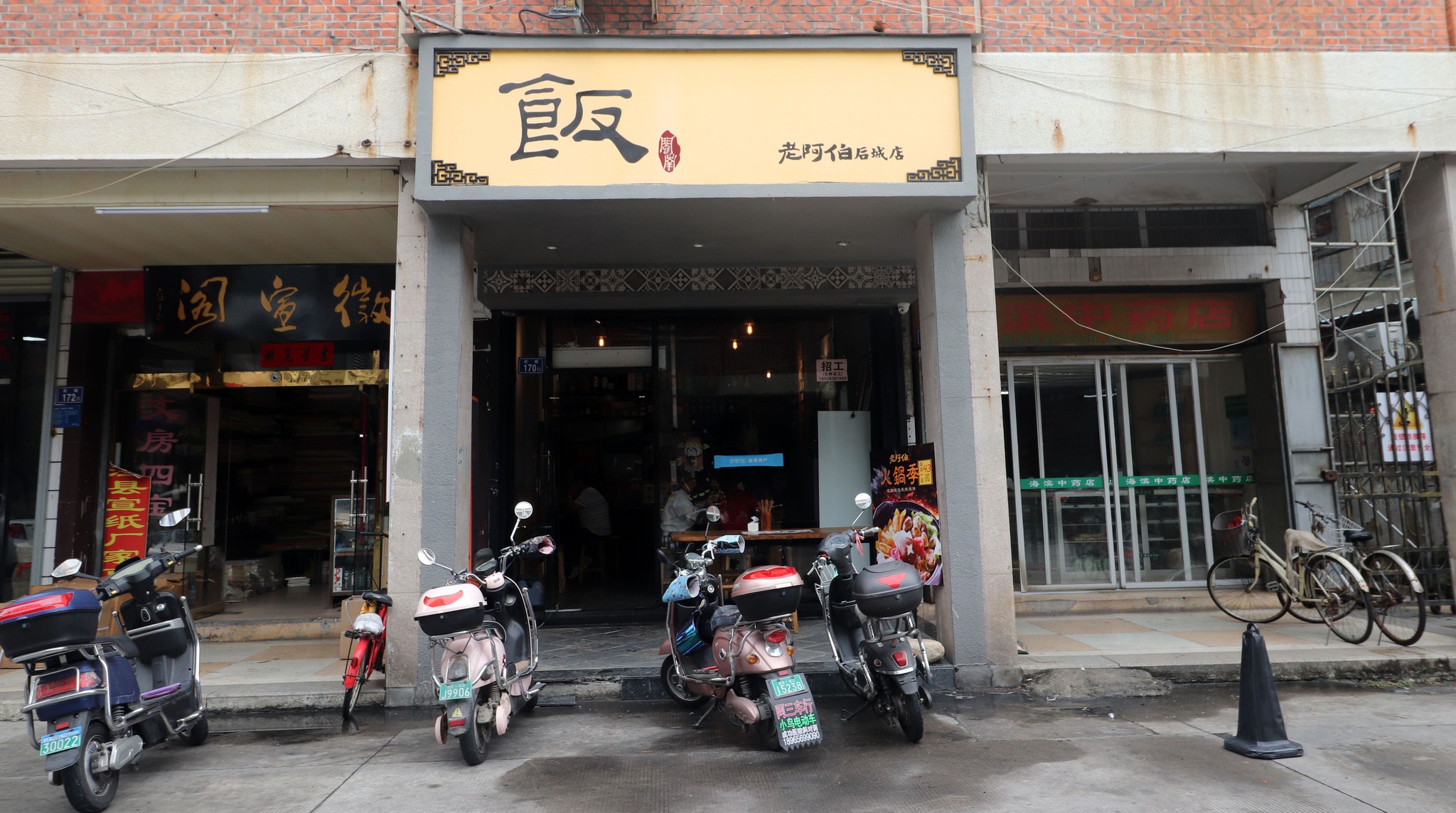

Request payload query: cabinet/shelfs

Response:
[329,495,376,609]
[1060,502,1205,543]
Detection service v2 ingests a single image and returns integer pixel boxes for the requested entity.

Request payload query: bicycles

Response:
[1292,498,1427,645]
[1204,497,1375,645]
[343,529,390,721]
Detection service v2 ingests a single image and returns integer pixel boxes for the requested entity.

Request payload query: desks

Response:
[670,526,874,541]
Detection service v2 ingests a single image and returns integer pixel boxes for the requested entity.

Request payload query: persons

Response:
[565,474,611,545]
[659,470,762,567]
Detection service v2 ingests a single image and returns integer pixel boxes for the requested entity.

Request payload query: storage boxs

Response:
[731,565,804,621]
[0,587,102,658]
[340,595,386,659]
[414,583,488,636]
[852,561,924,617]
[0,573,189,669]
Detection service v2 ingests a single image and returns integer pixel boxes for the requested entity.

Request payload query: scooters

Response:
[651,506,824,751]
[0,505,209,811]
[418,502,560,766]
[805,491,947,748]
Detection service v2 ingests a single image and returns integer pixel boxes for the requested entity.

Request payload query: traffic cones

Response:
[1220,621,1314,760]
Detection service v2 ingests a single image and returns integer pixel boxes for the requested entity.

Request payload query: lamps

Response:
[94,205,269,214]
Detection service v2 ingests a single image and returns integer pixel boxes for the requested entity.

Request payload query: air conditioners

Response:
[1337,321,1408,381]
